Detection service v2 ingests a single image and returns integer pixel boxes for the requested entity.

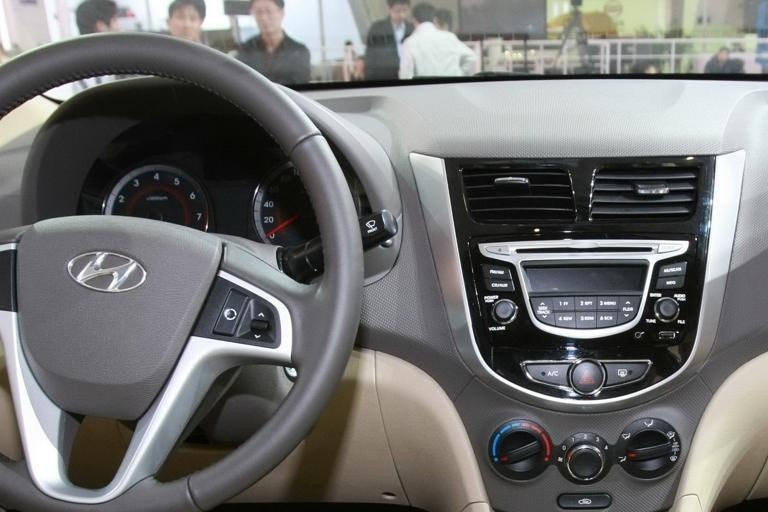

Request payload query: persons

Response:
[633,60,659,74]
[226,41,240,57]
[236,0,312,86]
[703,42,747,74]
[168,0,206,41]
[344,1,477,80]
[76,1,120,92]
